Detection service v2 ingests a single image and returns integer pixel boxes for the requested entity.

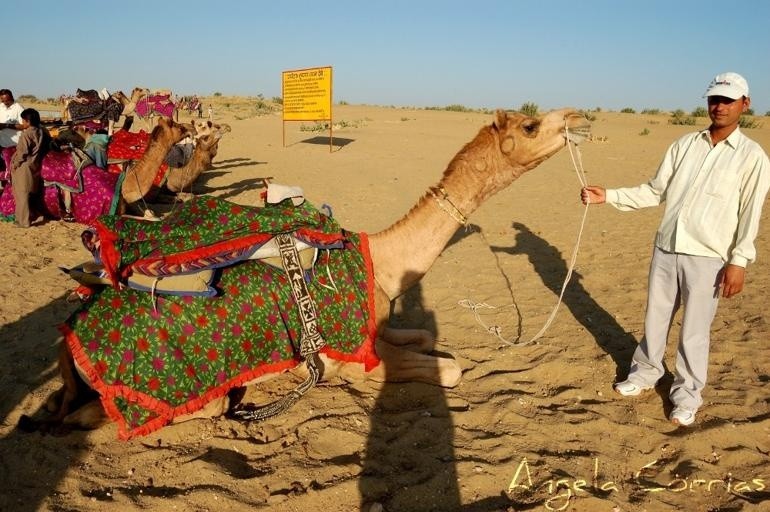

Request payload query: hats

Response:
[702,73,747,100]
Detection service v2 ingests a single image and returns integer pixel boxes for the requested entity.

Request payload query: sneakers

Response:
[669,407,697,426]
[614,379,654,396]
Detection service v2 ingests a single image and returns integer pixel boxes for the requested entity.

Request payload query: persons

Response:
[9,106,50,228]
[198,104,203,117]
[207,103,212,120]
[0,88,27,188]
[580,71,770,428]
